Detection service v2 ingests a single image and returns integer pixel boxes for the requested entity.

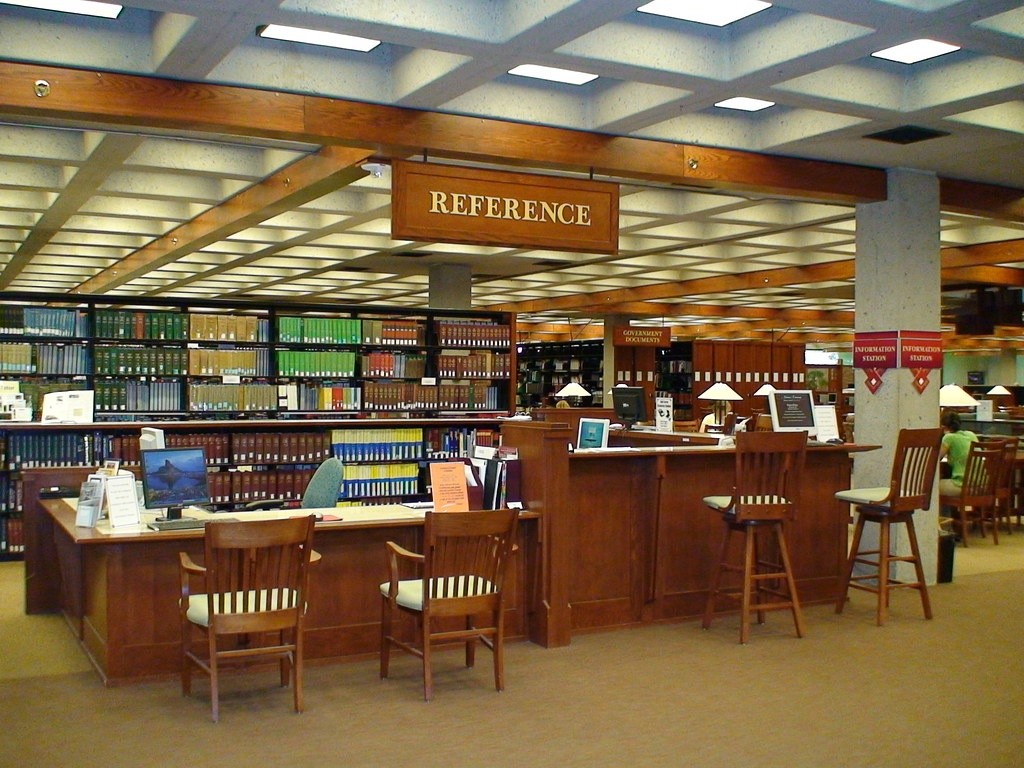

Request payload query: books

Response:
[0,308,91,422]
[94,311,190,422]
[362,319,438,419]
[279,316,361,420]
[0,517,25,555]
[1,428,510,513]
[188,313,277,421]
[439,321,511,419]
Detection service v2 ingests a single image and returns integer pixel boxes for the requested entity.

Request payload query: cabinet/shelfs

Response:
[734,341,755,432]
[654,340,714,427]
[713,341,735,412]
[515,338,635,422]
[0,290,518,421]
[791,342,805,390]
[771,342,791,390]
[0,418,515,562]
[753,342,772,432]
[634,346,655,421]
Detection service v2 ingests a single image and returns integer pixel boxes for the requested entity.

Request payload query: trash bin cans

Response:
[938,534,955,583]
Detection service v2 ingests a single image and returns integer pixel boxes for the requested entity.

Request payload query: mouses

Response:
[826,438,844,444]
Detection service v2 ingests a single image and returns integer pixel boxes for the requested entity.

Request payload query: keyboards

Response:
[807,441,829,447]
[146,518,240,532]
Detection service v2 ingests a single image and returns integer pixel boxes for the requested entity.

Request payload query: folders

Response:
[470,457,498,510]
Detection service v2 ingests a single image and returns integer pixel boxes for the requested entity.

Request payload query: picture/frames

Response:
[576,417,611,450]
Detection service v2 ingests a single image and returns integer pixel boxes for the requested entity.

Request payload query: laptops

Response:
[139,427,165,450]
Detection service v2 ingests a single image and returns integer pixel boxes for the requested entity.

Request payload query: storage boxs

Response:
[842,388,855,394]
[448,457,483,510]
[845,412,855,422]
[503,460,522,508]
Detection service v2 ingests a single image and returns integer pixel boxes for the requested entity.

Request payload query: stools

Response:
[702,430,809,646]
[834,428,944,628]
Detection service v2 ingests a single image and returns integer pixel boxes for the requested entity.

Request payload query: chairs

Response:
[177,512,318,724]
[746,408,765,432]
[704,414,738,435]
[938,440,1006,548]
[973,436,1020,535]
[245,457,345,511]
[754,413,774,431]
[377,507,522,703]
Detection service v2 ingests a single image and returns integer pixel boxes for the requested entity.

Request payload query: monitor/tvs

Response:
[140,446,211,521]
[577,418,610,449]
[768,389,819,436]
[828,393,837,403]
[612,387,648,431]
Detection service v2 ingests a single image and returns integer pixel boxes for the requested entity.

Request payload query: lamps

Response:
[752,383,779,415]
[697,382,744,425]
[985,385,1013,407]
[940,383,982,406]
[554,382,592,407]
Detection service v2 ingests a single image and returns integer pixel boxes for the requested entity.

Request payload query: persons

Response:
[939,412,989,496]
[699,401,732,433]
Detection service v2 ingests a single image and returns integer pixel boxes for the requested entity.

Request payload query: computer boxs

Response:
[817,394,832,405]
